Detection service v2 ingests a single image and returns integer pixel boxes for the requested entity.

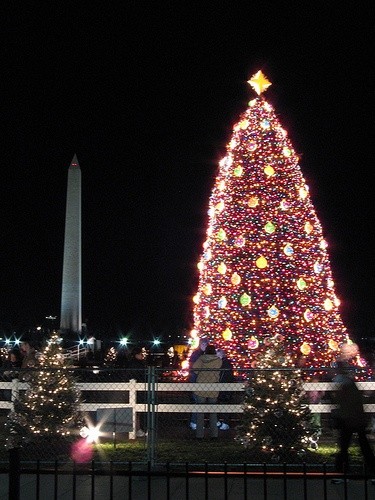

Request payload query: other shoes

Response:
[370,478,375,485]
[190,422,205,431]
[219,422,230,430]
[211,437,219,440]
[216,421,221,426]
[194,436,204,441]
[330,478,349,485]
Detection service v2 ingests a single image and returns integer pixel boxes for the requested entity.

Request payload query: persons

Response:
[188,338,230,440]
[330,359,375,460]
